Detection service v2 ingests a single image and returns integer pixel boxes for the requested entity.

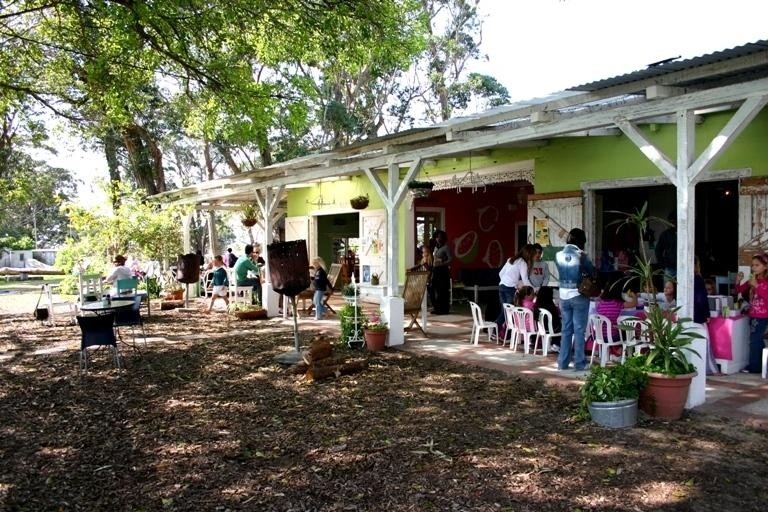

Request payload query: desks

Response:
[572,301,752,375]
[80,301,143,361]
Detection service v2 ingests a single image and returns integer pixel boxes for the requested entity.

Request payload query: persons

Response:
[734,252,767,373]
[343,250,355,277]
[421,245,434,305]
[430,230,453,315]
[703,278,717,295]
[694,256,725,376]
[490,228,678,371]
[100,255,161,300]
[196,244,265,313]
[312,258,328,320]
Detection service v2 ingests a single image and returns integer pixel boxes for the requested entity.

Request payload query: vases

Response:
[365,330,387,349]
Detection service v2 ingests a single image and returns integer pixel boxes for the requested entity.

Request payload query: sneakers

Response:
[551,344,562,353]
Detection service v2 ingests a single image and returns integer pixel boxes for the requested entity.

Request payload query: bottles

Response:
[102,295,107,307]
[107,294,111,306]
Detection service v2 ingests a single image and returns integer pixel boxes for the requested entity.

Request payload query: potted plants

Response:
[341,323,360,343]
[578,365,649,428]
[340,302,362,323]
[607,201,698,419]
[407,179,434,197]
[349,193,368,210]
[341,283,360,299]
[239,200,260,227]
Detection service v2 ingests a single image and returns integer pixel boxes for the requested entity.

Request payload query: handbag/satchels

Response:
[579,255,602,298]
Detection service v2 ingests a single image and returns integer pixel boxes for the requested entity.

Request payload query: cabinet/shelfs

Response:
[343,272,366,348]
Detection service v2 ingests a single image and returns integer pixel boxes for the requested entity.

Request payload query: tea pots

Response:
[371,271,384,286]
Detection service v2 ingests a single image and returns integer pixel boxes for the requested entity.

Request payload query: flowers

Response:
[365,310,387,331]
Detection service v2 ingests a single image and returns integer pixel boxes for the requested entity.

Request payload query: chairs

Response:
[534,308,569,357]
[617,315,656,366]
[112,296,148,353]
[76,312,120,376]
[510,307,544,355]
[200,269,220,299]
[469,301,499,345]
[138,275,150,318]
[38,284,72,324]
[229,268,254,310]
[449,278,469,310]
[503,303,532,350]
[299,263,343,316]
[402,271,431,338]
[71,274,103,322]
[104,279,138,307]
[589,314,624,369]
